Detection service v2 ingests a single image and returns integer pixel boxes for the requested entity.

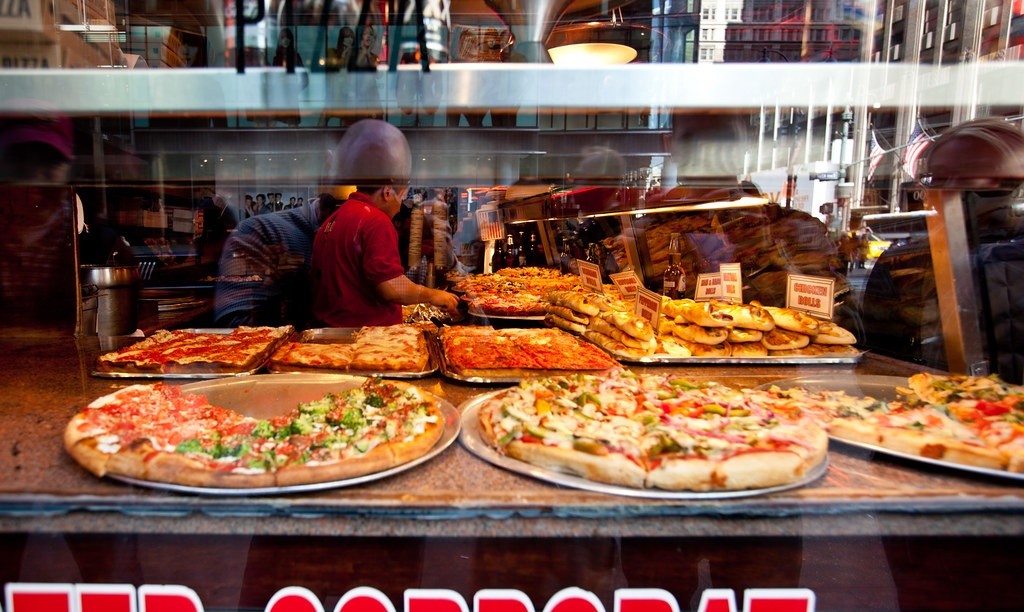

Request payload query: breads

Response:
[544,283,859,357]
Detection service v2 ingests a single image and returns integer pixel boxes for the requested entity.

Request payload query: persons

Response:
[244,192,303,218]
[316,24,384,125]
[309,180,461,326]
[215,119,487,329]
[272,28,308,127]
[561,106,1024,383]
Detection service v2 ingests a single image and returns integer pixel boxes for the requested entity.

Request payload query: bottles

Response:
[560,235,573,274]
[586,243,601,265]
[554,218,606,256]
[682,232,712,279]
[492,231,540,274]
[663,233,686,300]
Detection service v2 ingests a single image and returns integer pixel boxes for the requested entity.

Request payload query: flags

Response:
[868,132,884,180]
[901,120,932,178]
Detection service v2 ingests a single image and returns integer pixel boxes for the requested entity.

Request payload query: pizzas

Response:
[770,374,1024,472]
[603,207,850,307]
[445,266,584,317]
[477,371,829,490]
[269,324,429,373]
[97,326,295,373]
[438,324,621,375]
[64,376,446,488]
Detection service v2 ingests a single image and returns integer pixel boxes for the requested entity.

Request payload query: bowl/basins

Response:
[87,265,142,289]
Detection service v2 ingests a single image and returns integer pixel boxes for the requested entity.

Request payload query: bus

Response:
[859,210,939,260]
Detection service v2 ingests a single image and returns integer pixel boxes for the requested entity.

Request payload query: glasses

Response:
[920,172,1024,198]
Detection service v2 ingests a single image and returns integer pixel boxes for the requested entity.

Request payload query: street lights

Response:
[759,45,837,213]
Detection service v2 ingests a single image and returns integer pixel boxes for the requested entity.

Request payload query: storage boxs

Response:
[145,59,171,68]
[123,27,182,55]
[0,0,130,69]
[119,42,186,68]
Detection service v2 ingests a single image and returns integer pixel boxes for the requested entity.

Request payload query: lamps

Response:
[499,23,668,65]
